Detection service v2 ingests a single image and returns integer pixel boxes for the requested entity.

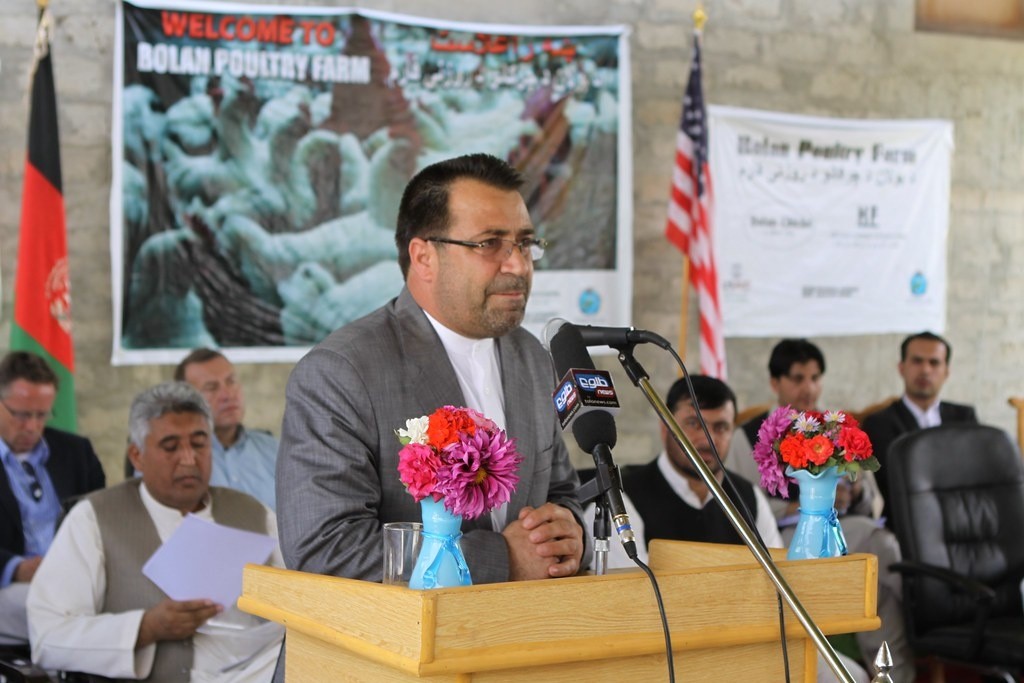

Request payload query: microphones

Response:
[542,318,672,351]
[550,327,639,559]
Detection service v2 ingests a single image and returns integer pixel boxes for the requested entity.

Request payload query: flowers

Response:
[396,404,529,520]
[753,404,883,505]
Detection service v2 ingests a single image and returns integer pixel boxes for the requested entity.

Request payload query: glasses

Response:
[424,237,548,265]
[0,398,49,419]
[21,461,43,502]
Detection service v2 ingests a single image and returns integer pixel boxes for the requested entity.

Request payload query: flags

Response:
[7,7,77,434]
[664,26,727,381]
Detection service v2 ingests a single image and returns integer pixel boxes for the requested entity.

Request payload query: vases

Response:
[407,495,475,588]
[785,462,857,565]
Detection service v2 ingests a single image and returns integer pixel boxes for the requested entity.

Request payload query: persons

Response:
[27,381,286,683]
[585,374,869,683]
[276,153,594,583]
[737,336,914,683]
[0,351,106,646]
[860,333,980,533]
[132,344,280,512]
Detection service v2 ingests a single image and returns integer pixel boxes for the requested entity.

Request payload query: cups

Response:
[382,522,424,585]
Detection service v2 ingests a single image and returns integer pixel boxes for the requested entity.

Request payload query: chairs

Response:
[886,422,1024,683]
[737,403,771,446]
